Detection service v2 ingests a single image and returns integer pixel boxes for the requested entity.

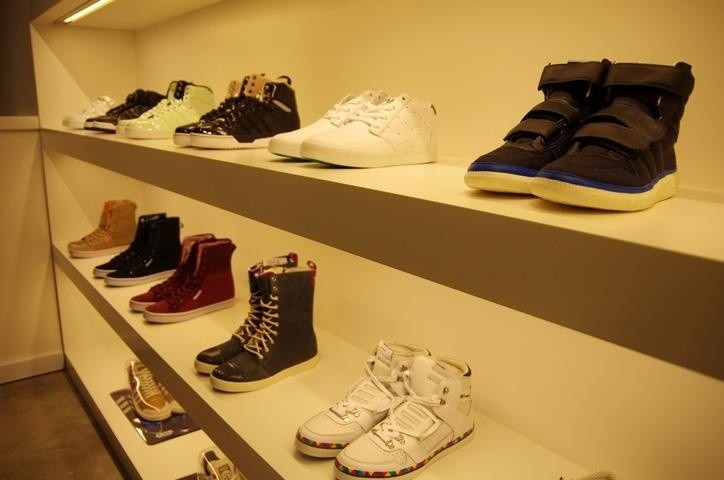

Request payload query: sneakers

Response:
[128,362,187,423]
[292,338,479,479]
[62,95,113,131]
[114,80,216,140]
[173,72,302,151]
[84,87,168,133]
[195,445,241,480]
[265,91,440,169]
[464,57,696,214]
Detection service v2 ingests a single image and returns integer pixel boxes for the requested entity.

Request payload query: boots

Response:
[93,212,183,289]
[128,233,237,324]
[66,198,137,258]
[194,251,319,395]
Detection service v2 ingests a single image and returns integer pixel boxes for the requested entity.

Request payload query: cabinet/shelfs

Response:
[27,0,724,480]
